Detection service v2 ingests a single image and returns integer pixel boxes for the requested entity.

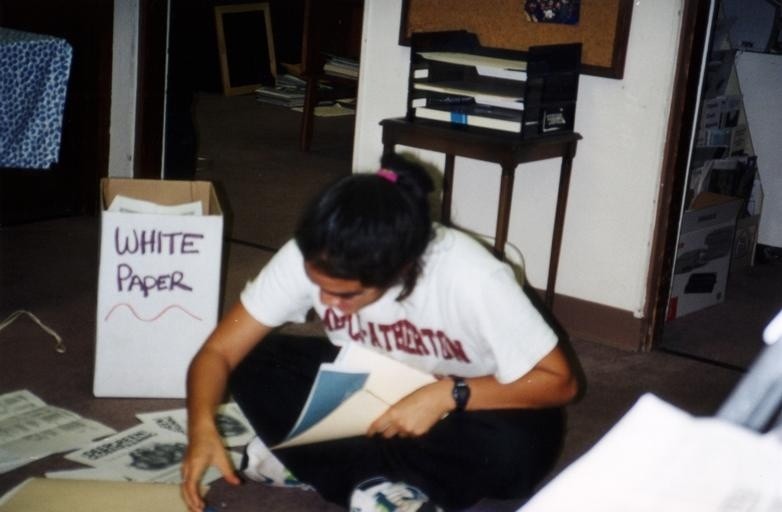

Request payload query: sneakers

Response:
[353,483,444,512]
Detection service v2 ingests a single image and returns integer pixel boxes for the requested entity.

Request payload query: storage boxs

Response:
[93,178,225,401]
[665,191,743,321]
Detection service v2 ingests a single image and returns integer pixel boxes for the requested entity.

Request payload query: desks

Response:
[379,112,582,325]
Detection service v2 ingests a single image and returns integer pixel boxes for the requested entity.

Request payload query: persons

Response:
[176,149,584,511]
[523,1,580,26]
[251,41,360,118]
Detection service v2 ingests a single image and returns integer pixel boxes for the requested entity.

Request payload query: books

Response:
[415,50,528,84]
[412,81,524,111]
[412,104,529,138]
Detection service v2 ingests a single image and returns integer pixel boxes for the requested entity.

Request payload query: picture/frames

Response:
[400,1,634,82]
[217,4,281,99]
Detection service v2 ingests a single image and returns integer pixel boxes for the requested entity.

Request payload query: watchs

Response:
[445,374,470,423]
